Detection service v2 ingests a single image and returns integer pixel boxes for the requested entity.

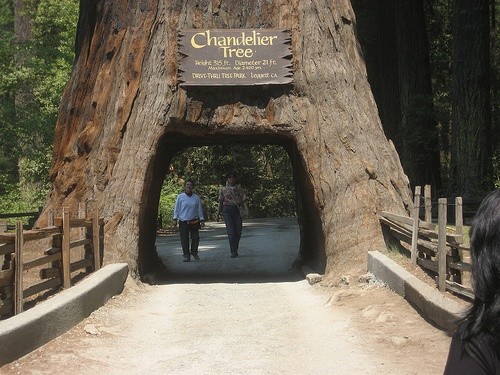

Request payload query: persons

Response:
[173,180,205,262]
[443,188,500,375]
[216,172,249,258]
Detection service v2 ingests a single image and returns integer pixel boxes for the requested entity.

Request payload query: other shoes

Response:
[182,255,201,262]
[229,252,239,258]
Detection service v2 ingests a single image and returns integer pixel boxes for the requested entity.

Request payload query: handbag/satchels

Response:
[238,202,249,218]
